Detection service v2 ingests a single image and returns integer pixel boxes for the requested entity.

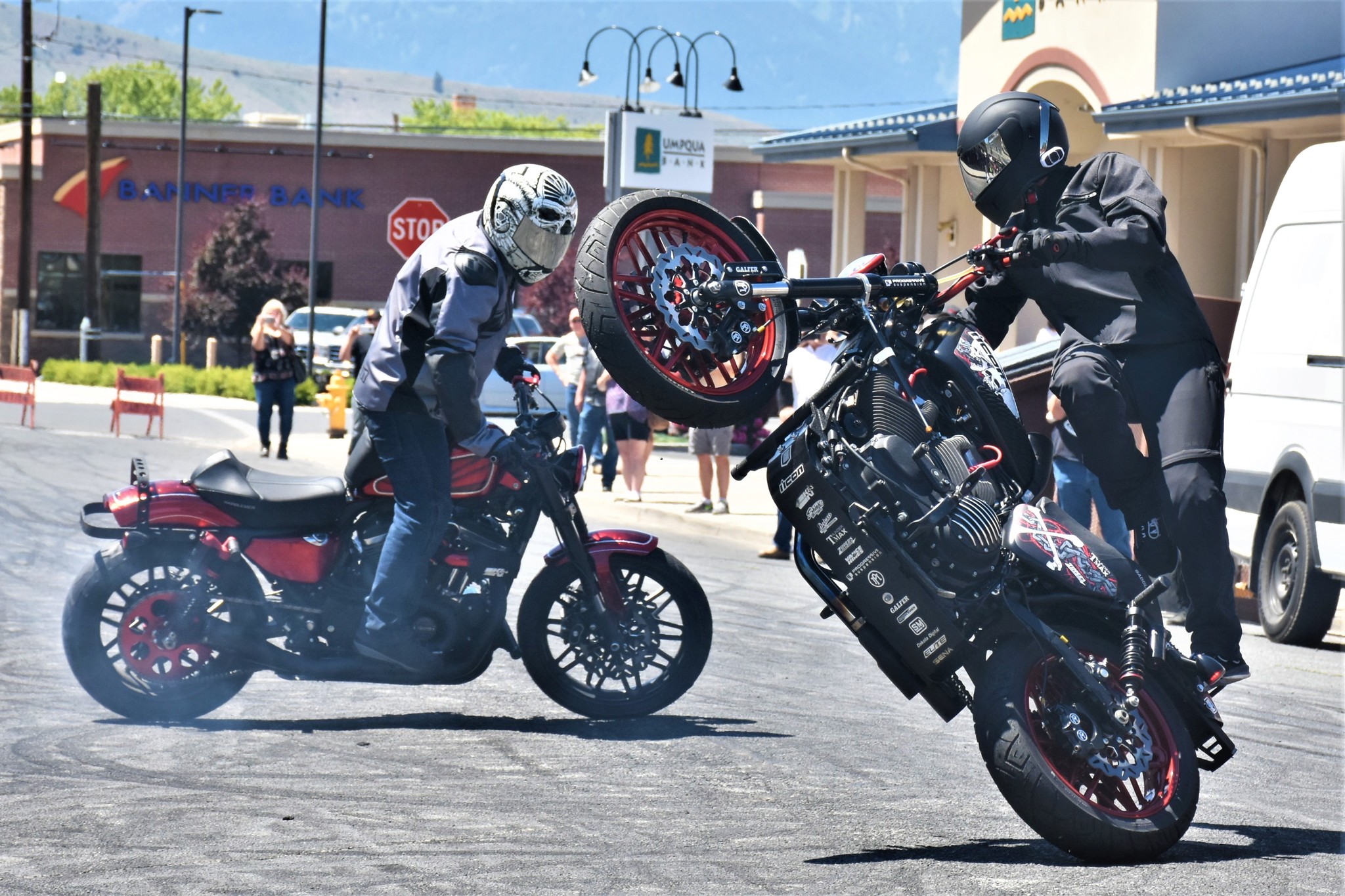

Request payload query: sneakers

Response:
[353,633,443,672]
[1132,510,1180,579]
[1188,652,1251,688]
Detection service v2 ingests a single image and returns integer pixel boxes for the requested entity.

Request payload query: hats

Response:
[569,308,579,321]
[364,308,382,318]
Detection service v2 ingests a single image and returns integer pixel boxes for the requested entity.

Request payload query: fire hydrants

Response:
[313,368,351,439]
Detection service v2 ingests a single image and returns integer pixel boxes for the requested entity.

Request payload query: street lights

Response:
[170,6,222,364]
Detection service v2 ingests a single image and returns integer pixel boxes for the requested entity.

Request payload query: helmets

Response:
[956,91,1070,229]
[482,164,578,286]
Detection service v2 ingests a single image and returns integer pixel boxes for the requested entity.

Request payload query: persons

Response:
[596,368,651,502]
[685,425,734,514]
[1166,548,1191,626]
[952,90,1253,683]
[574,340,619,491]
[353,162,578,673]
[339,308,383,458]
[1047,363,1146,563]
[545,308,621,474]
[759,332,840,561]
[250,299,294,460]
[644,409,654,476]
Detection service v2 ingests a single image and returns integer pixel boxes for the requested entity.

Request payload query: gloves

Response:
[1012,227,1068,266]
[485,435,530,483]
[495,351,541,384]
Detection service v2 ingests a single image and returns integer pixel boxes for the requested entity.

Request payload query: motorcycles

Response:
[61,372,714,727]
[572,186,1237,864]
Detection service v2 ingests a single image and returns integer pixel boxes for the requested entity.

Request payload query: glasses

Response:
[368,317,380,321]
[573,320,580,322]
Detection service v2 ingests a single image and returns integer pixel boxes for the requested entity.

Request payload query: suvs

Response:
[283,305,384,364]
[506,310,544,338]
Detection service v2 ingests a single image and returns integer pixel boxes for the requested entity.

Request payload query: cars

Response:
[478,335,568,417]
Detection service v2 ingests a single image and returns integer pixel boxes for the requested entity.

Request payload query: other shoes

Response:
[593,465,603,474]
[278,447,288,459]
[757,546,790,559]
[260,448,269,456]
[712,501,730,515]
[616,490,641,502]
[686,501,713,513]
[603,485,612,491]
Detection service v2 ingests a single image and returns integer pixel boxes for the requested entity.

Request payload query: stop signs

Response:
[385,196,450,261]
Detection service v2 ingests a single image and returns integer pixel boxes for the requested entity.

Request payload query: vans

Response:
[1221,140,1345,646]
